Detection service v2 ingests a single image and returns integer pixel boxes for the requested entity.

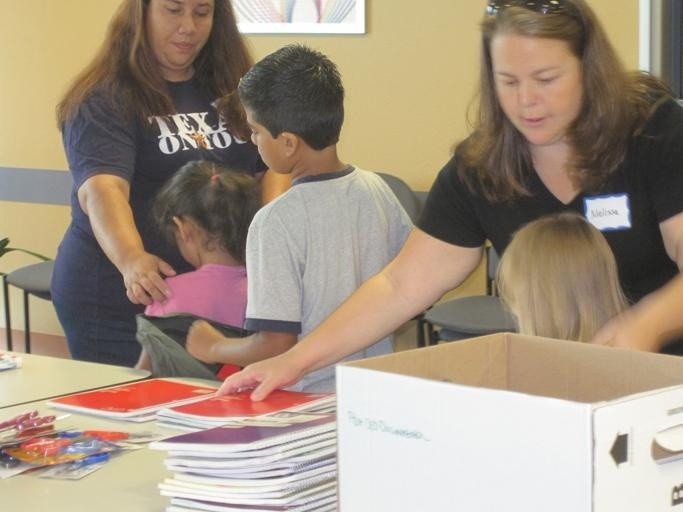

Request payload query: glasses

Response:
[485,1,566,16]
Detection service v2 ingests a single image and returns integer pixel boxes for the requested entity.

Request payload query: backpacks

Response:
[134,313,248,379]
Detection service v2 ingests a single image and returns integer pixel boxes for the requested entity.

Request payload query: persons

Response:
[214,0,682,407]
[46,0,291,369]
[491,212,629,346]
[127,153,266,384]
[179,41,421,395]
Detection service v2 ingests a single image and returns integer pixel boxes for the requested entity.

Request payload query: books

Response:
[147,386,342,512]
[47,378,216,424]
[0,351,21,372]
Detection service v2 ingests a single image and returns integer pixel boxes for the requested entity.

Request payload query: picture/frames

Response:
[231,0,366,34]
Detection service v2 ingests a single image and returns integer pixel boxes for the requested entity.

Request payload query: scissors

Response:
[0,410,129,475]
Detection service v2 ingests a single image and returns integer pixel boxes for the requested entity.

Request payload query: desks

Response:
[0,349,152,419]
[0,376,222,512]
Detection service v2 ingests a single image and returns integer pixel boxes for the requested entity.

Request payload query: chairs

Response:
[4,261,55,354]
[425,244,519,340]
[378,171,421,224]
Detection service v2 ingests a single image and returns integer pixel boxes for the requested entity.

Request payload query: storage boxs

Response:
[335,331,683,512]
